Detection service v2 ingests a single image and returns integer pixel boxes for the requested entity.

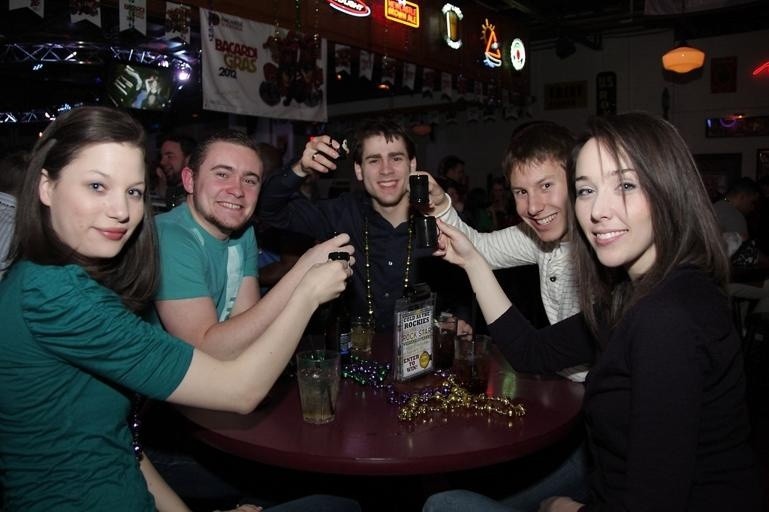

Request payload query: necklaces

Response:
[362,217,413,331]
[132,391,142,466]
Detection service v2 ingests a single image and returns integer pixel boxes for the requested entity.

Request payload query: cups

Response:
[409,173,430,208]
[353,317,373,353]
[453,332,495,395]
[434,316,456,375]
[412,216,440,254]
[328,251,350,267]
[320,229,341,242]
[320,132,353,179]
[296,349,341,424]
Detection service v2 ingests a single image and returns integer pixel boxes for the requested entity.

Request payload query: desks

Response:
[175,322,586,511]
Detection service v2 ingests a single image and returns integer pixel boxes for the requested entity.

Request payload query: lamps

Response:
[659,2,706,73]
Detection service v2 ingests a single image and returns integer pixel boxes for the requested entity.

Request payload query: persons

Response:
[702,169,767,351]
[142,131,356,361]
[437,156,522,239]
[254,118,447,334]
[403,116,608,391]
[420,111,750,512]
[2,105,352,511]
